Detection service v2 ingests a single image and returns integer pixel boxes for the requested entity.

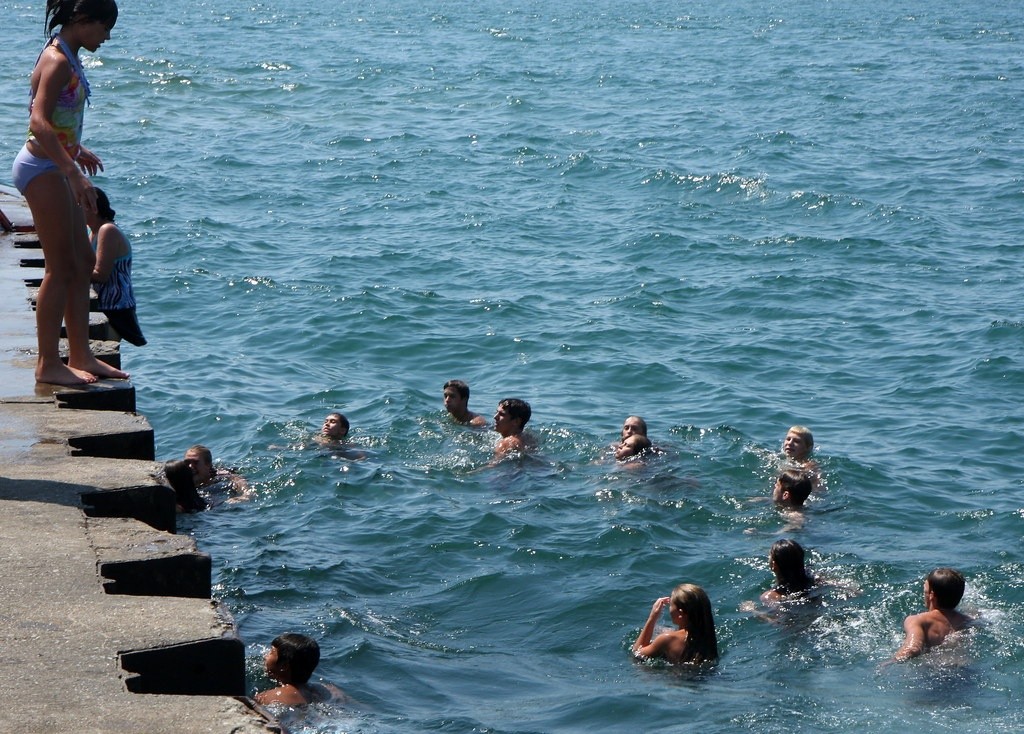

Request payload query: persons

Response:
[461,396,554,480]
[627,581,722,677]
[10,0,131,386]
[252,632,375,716]
[294,411,366,463]
[597,414,669,476]
[873,562,988,705]
[431,374,490,428]
[776,424,824,493]
[739,530,863,631]
[743,467,819,539]
[163,439,259,511]
[81,185,148,348]
